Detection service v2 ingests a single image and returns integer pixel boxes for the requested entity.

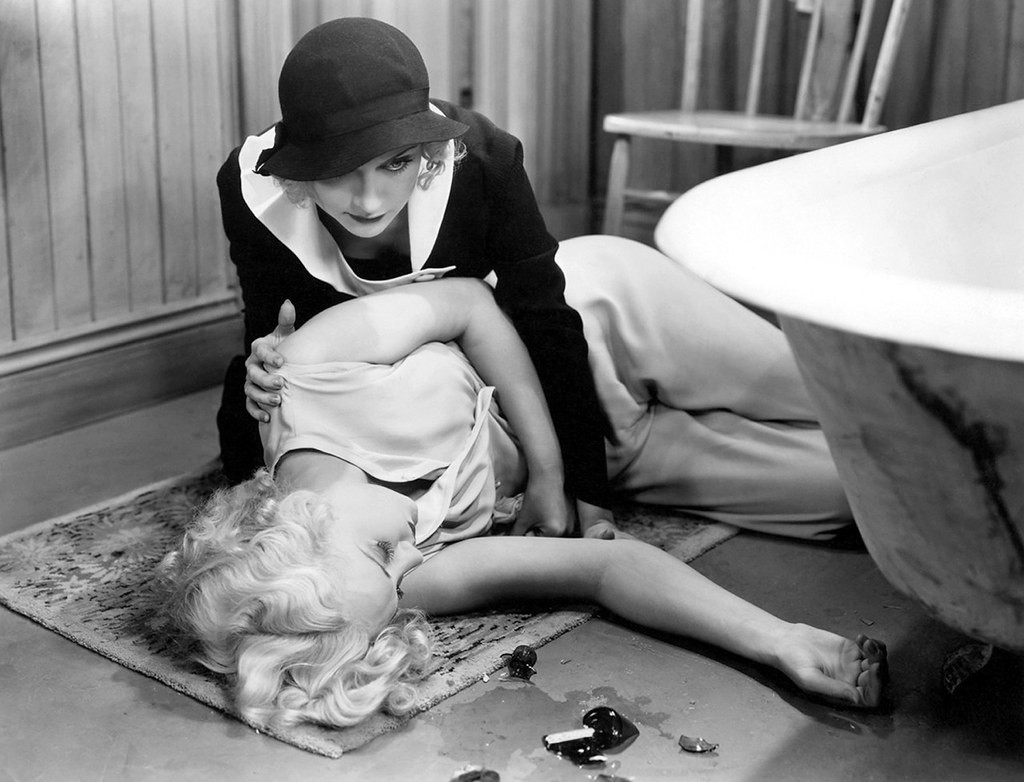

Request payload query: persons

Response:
[216,18,645,541]
[151,233,887,734]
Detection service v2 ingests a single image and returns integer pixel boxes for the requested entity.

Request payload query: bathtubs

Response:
[655,97,1022,659]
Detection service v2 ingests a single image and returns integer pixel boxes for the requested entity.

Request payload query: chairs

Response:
[601,0,910,249]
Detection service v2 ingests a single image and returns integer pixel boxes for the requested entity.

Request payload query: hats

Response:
[252,16,469,182]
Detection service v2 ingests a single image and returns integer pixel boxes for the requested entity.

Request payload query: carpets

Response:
[0,451,741,763]
